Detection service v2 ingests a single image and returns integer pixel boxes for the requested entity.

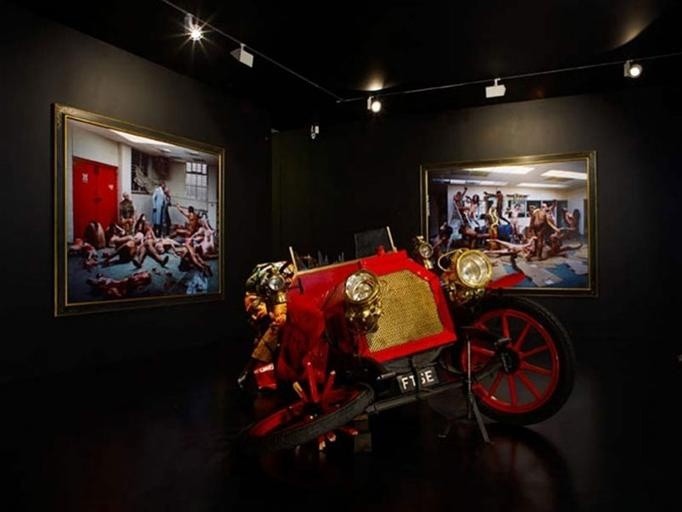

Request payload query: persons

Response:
[65,176,218,299]
[430,185,581,277]
[243,289,289,363]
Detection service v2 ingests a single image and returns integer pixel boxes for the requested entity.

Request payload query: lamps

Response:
[622,60,645,80]
[367,94,382,113]
[484,78,507,99]
[230,43,254,68]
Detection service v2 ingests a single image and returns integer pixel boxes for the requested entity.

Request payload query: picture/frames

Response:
[419,149,602,299]
[51,103,227,320]
